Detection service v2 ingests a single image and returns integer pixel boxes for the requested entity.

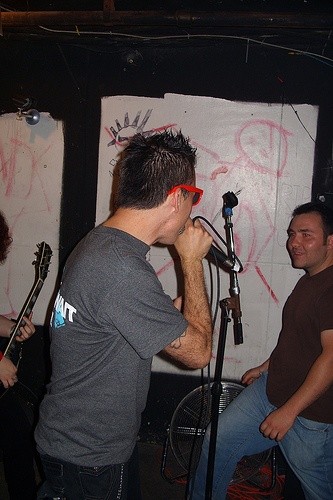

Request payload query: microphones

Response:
[178,226,242,272]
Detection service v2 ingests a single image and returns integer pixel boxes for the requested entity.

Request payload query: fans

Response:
[162,382,278,491]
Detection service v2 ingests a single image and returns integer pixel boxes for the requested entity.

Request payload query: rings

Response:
[12,376,16,380]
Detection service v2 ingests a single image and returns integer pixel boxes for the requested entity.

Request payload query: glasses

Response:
[167,184,203,206]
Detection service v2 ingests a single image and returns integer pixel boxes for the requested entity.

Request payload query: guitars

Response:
[0,242,51,395]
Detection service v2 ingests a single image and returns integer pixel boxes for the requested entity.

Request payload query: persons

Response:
[0,213,36,389]
[186,201,333,500]
[33,128,213,500]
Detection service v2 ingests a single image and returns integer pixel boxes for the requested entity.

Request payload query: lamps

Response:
[16,101,42,126]
